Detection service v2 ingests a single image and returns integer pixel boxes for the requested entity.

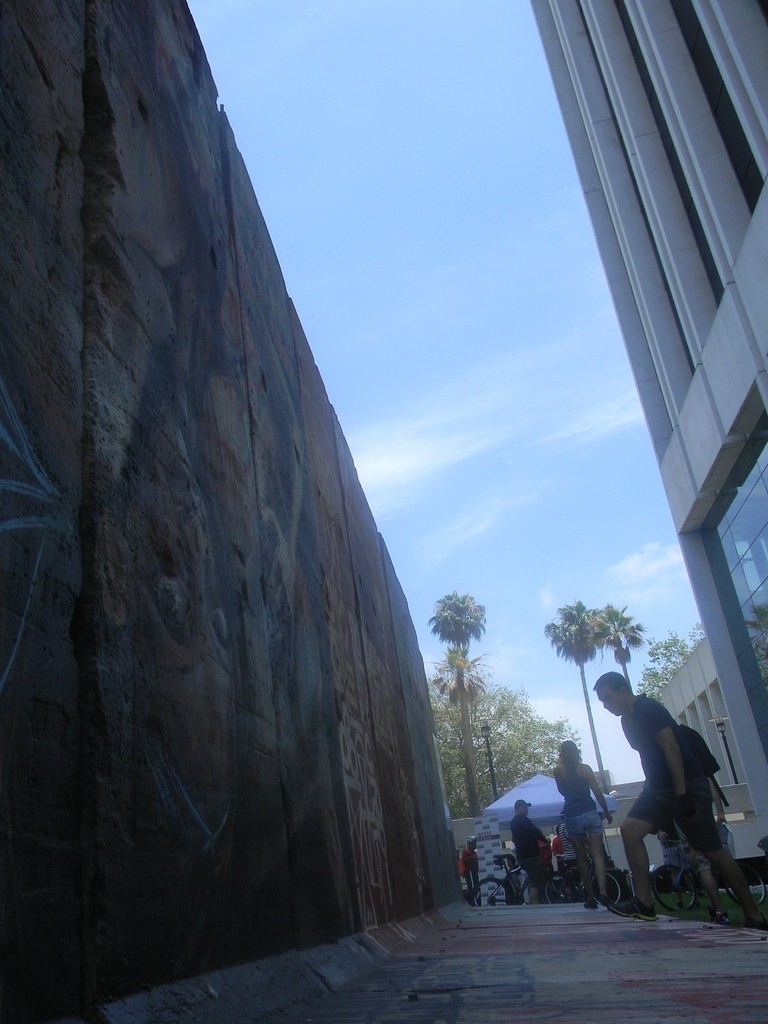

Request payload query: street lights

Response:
[481,725,498,801]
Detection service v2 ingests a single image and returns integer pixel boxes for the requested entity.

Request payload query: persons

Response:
[593,672,768,931]
[461,835,482,908]
[505,741,614,910]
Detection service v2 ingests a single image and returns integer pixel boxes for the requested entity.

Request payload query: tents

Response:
[484,773,616,859]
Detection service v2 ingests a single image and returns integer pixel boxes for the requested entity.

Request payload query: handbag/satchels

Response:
[659,725,721,792]
[538,839,552,863]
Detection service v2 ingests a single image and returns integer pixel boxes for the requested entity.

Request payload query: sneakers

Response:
[607,896,657,921]
[744,912,768,931]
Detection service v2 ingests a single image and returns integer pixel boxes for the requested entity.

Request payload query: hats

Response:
[515,800,531,811]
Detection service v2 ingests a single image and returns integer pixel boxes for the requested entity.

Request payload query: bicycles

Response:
[652,838,766,914]
[472,855,532,908]
[545,855,621,906]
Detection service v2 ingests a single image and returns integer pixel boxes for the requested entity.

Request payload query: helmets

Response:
[464,835,477,843]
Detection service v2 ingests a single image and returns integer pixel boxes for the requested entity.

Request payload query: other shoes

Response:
[584,901,597,908]
[675,901,683,908]
[713,910,731,925]
[693,901,700,907]
